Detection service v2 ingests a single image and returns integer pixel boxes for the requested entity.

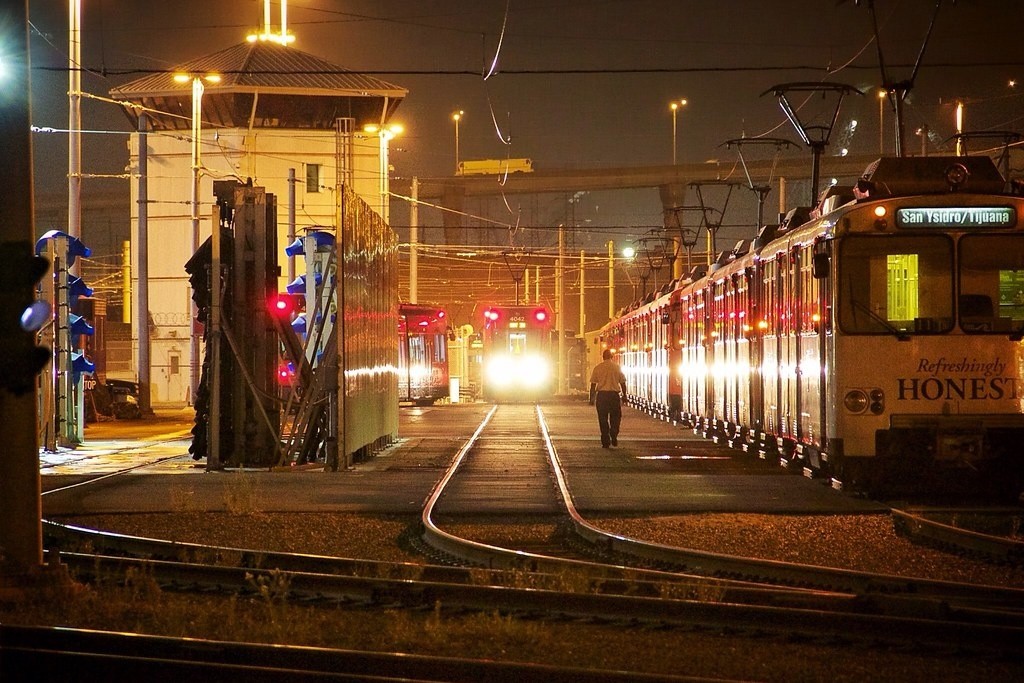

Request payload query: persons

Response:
[589,349,627,447]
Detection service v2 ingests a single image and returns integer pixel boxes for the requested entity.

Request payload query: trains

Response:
[563,79,1024,497]
[476,250,557,404]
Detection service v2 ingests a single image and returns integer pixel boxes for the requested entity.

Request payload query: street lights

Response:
[453,110,464,177]
[879,90,887,153]
[175,71,222,409]
[669,96,689,166]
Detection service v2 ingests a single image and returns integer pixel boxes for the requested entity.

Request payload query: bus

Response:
[454,157,535,176]
[271,287,449,415]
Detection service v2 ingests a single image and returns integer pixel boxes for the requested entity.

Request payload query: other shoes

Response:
[613,439,618,446]
[602,442,610,449]
